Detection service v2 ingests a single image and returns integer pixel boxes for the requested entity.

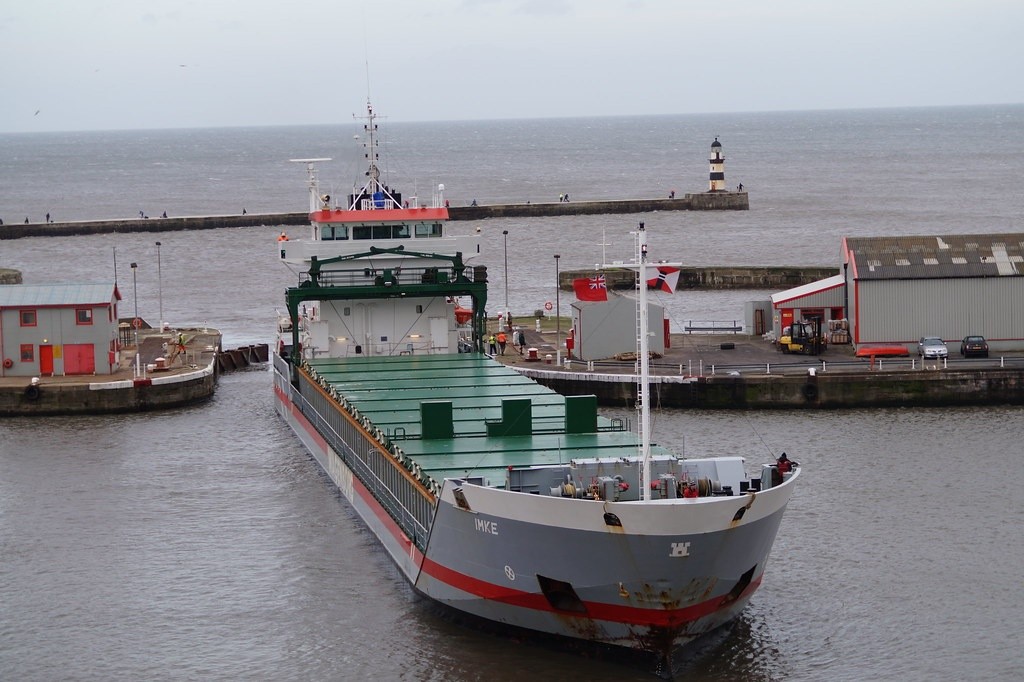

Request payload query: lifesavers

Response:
[803,383,818,398]
[133,319,142,328]
[545,302,553,311]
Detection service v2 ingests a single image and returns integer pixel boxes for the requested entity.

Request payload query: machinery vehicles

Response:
[778,315,829,356]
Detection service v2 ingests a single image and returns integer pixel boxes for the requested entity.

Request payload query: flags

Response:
[574,274,608,301]
[646,266,680,293]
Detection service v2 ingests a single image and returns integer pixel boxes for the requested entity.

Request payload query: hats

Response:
[281,233,286,237]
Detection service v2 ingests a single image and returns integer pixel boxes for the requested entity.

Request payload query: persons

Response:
[684,483,698,498]
[671,190,675,198]
[489,335,497,355]
[739,183,743,192]
[564,193,569,202]
[778,453,798,484]
[498,332,507,356]
[178,333,186,355]
[559,192,563,202]
[446,199,449,206]
[472,199,476,205]
[519,330,526,354]
[405,201,409,208]
[507,311,513,331]
[278,233,288,241]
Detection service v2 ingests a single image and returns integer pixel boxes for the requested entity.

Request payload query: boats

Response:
[268,79,808,681]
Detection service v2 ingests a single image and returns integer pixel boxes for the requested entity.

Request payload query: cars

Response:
[917,336,948,359]
[960,335,989,359]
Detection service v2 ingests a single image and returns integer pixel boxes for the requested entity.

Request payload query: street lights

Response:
[502,230,508,322]
[553,254,562,366]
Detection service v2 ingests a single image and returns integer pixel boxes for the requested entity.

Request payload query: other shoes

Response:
[177,352,180,354]
[501,353,505,356]
[520,348,522,350]
[183,352,186,354]
[521,353,524,355]
